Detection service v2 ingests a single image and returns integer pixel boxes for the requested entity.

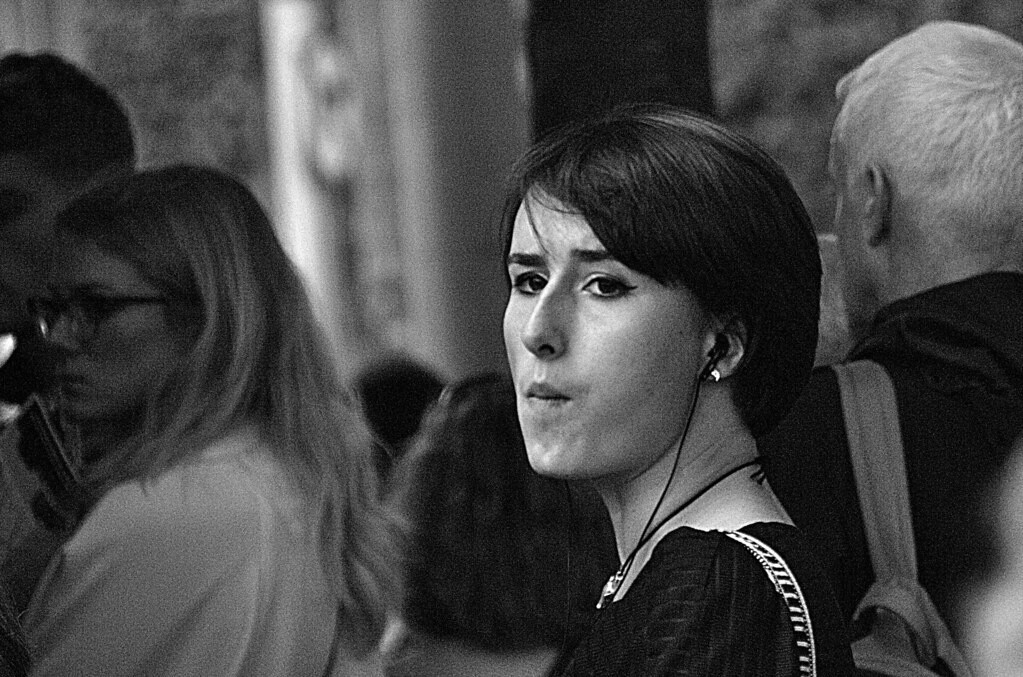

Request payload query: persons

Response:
[495,105,860,677]
[778,19,1023,677]
[0,47,616,677]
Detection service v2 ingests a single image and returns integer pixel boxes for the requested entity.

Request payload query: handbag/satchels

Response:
[832,359,973,677]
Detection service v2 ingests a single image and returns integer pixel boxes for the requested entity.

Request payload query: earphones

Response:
[699,332,729,382]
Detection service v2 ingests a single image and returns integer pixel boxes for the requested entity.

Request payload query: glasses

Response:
[25,291,170,342]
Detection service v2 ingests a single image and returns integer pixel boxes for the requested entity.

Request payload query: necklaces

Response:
[594,458,770,608]
